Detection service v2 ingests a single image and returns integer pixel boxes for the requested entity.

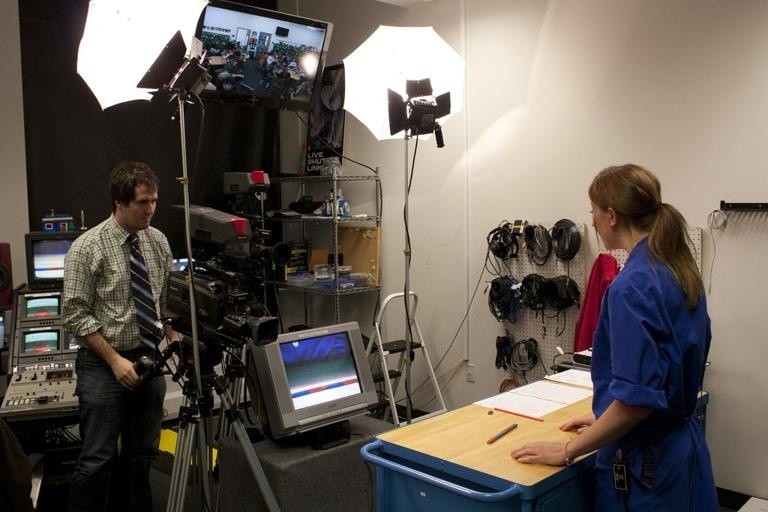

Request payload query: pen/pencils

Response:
[487,423,518,444]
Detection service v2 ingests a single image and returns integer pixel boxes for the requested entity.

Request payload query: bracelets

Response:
[564,441,574,466]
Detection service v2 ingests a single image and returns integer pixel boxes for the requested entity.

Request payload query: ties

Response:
[127,234,164,351]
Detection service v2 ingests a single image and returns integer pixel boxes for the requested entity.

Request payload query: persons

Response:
[510,163,718,512]
[60,162,183,512]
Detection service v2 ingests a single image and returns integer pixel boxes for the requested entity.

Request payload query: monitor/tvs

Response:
[188,0,334,112]
[247,321,380,450]
[14,326,62,357]
[16,292,62,321]
[24,231,84,290]
[169,258,196,272]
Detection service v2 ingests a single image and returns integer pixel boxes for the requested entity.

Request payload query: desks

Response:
[358,370,709,512]
[217,414,397,512]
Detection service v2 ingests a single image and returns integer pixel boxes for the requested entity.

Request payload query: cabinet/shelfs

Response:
[262,164,381,346]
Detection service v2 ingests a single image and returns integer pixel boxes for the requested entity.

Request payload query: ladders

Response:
[366,291,446,427]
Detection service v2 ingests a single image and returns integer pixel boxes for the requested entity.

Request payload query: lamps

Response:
[137,30,214,512]
[342,25,466,424]
